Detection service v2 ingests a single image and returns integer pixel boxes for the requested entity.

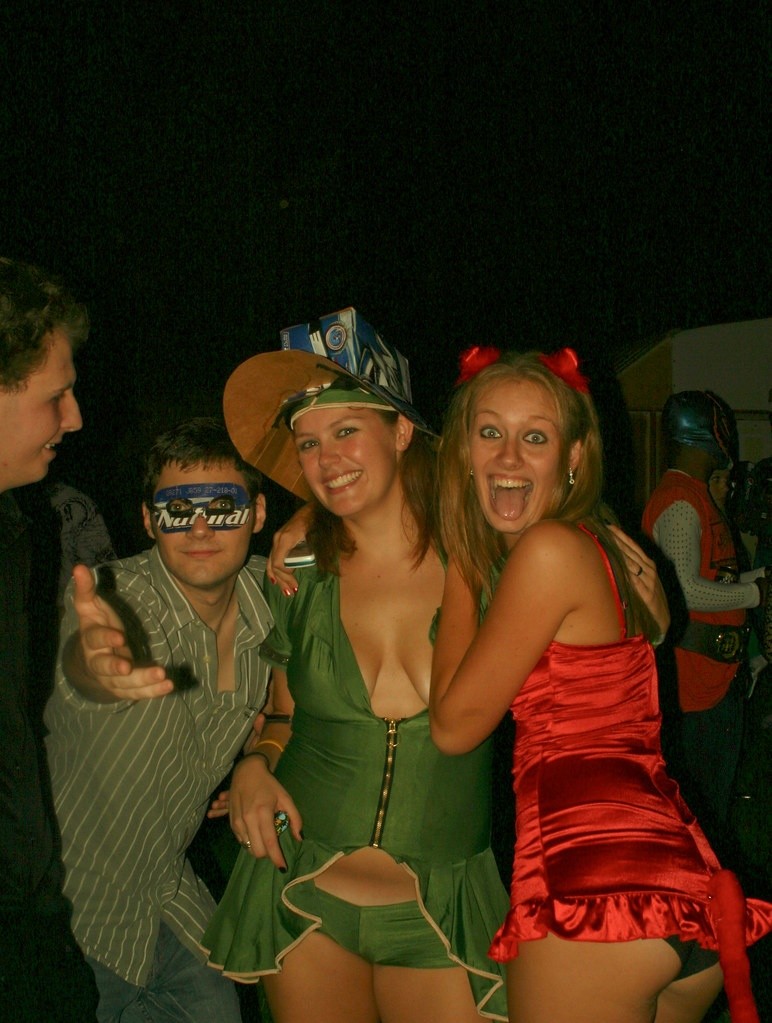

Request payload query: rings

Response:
[242,841,252,849]
[635,567,642,576]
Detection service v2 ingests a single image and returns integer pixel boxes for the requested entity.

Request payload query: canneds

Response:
[715,565,739,584]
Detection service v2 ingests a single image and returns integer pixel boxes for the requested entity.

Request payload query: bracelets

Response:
[242,752,270,769]
[254,739,284,752]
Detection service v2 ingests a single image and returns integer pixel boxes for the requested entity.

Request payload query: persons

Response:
[202,347,670,1023]
[427,346,772,1023]
[642,390,772,855]
[0,256,91,1023]
[39,414,277,1023]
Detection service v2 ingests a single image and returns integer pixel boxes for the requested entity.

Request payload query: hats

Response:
[663,389,736,472]
[220,308,437,504]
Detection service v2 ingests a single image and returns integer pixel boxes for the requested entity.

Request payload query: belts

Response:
[671,618,751,664]
